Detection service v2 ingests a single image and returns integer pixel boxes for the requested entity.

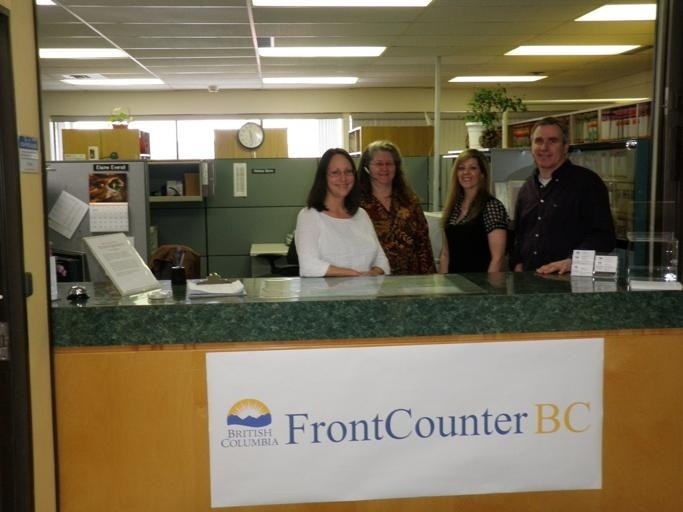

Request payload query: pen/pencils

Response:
[175,251,185,269]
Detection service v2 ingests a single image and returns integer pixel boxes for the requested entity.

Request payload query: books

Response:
[494,179,525,221]
[508,122,531,148]
[601,104,649,140]
[572,111,597,143]
[82,233,161,297]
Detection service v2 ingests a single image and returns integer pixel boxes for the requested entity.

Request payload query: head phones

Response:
[360,165,371,178]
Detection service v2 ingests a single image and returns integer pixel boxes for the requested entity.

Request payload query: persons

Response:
[294,148,391,277]
[358,140,436,276]
[508,117,615,277]
[439,148,511,274]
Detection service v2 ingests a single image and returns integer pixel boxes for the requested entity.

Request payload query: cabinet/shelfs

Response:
[353,155,428,212]
[206,159,309,272]
[508,103,651,243]
[348,125,434,156]
[145,160,205,281]
[428,157,449,213]
[62,129,140,161]
[214,129,289,160]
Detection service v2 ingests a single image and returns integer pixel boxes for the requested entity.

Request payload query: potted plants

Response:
[465,110,486,149]
[463,82,528,149]
[109,104,129,129]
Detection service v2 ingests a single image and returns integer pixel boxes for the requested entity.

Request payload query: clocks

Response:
[237,122,265,149]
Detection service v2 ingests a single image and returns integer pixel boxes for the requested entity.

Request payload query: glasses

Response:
[363,158,398,170]
[325,165,356,178]
